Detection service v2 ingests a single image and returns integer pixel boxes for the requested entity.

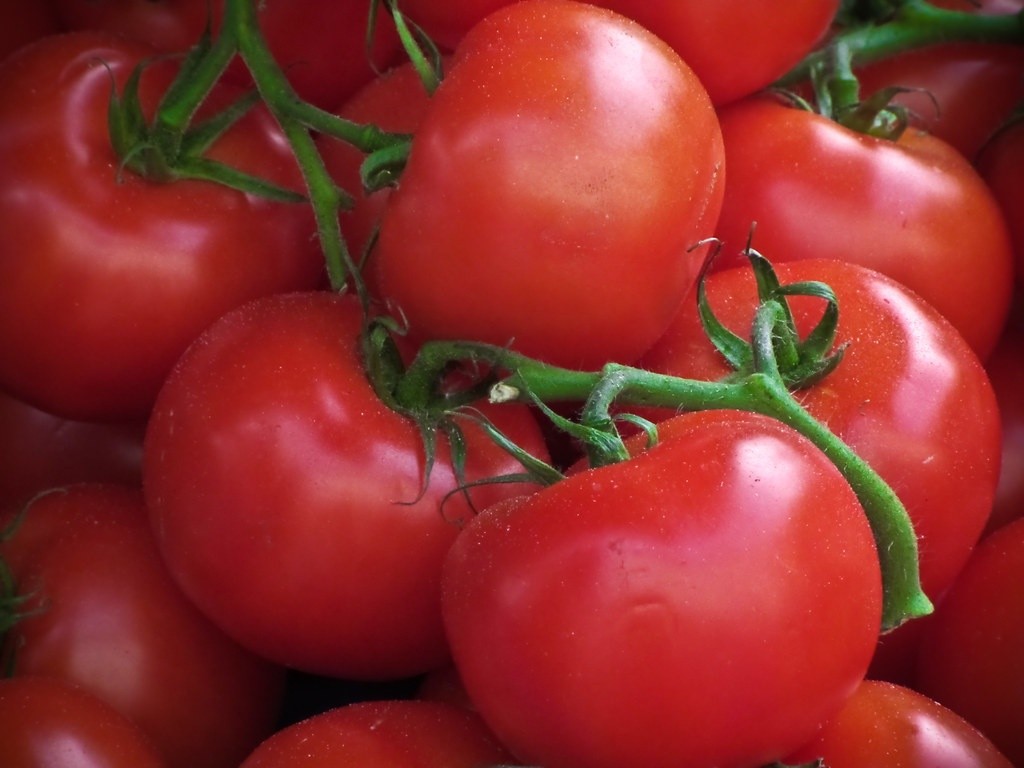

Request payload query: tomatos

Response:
[0,1,1024,768]
